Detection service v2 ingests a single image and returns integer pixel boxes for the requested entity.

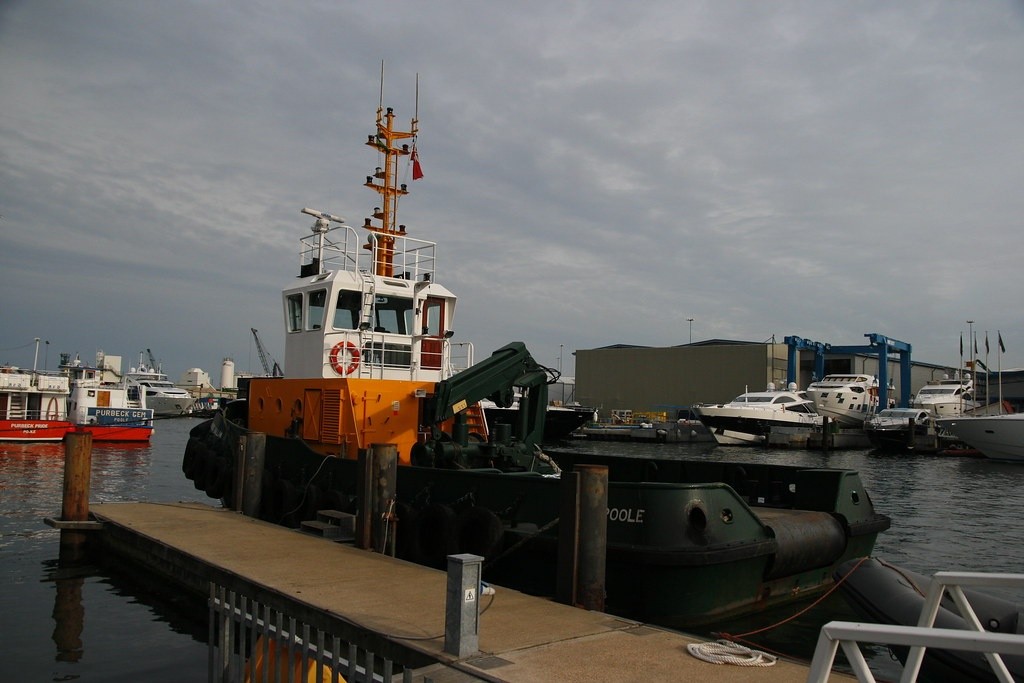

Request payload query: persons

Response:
[890,401,894,408]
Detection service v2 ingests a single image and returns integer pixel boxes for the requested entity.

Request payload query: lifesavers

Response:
[329,340,360,376]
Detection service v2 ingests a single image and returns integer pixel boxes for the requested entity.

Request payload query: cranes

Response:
[250,328,284,376]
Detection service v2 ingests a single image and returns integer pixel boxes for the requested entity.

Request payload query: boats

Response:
[182,57,893,634]
[833,556,1024,683]
[0,338,198,443]
[690,370,1024,463]
[478,393,595,439]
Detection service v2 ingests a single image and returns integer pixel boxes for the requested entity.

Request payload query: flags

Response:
[960,337,963,355]
[410,151,424,180]
[975,339,978,353]
[985,337,989,353]
[999,334,1006,353]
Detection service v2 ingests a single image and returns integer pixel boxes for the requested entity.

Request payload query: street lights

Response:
[967,320,974,362]
[557,344,563,373]
[686,318,695,344]
